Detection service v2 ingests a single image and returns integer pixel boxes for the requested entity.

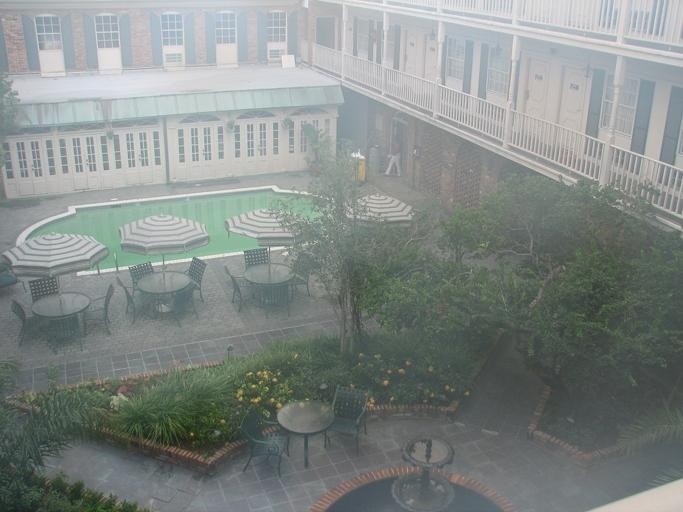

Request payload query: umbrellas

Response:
[343,194,414,230]
[2,230,108,306]
[116,213,208,288]
[224,209,311,269]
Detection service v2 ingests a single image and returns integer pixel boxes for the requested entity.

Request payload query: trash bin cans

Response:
[351,151,366,185]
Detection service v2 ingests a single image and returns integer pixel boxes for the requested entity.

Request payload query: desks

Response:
[273,397,335,469]
[31,293,90,332]
[136,270,192,314]
[244,263,293,301]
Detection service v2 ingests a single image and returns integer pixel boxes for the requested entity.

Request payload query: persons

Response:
[383,134,403,176]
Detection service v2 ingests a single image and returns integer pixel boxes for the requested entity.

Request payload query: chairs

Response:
[221,267,256,312]
[116,276,146,324]
[127,262,158,302]
[46,313,82,351]
[163,282,196,326]
[178,256,208,302]
[28,278,59,304]
[262,284,292,319]
[237,407,291,477]
[241,248,275,272]
[286,258,309,297]
[84,283,115,336]
[321,384,371,458]
[11,300,42,347]
